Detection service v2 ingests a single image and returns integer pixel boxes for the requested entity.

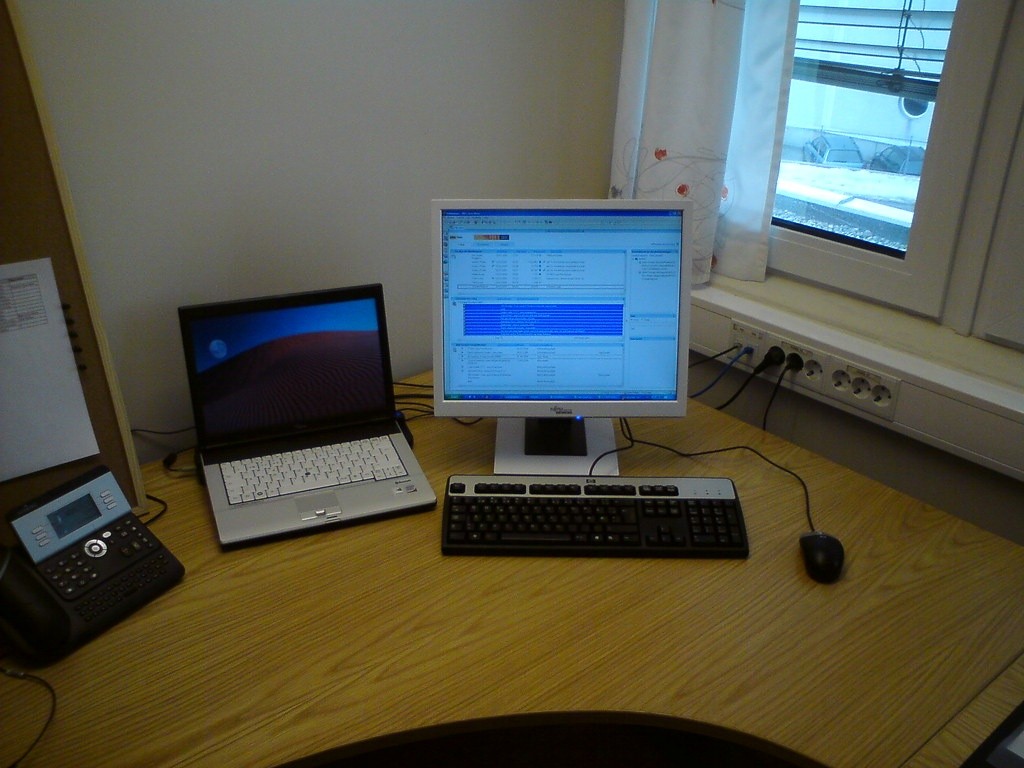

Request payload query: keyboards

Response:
[441,474,750,558]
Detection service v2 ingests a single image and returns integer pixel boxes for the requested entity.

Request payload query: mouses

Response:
[799,530,845,583]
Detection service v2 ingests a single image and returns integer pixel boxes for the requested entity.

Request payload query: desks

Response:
[0,369,1024,768]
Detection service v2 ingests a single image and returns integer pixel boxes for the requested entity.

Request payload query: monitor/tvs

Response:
[429,198,694,476]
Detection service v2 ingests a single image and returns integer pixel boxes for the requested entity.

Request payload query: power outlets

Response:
[725,320,899,422]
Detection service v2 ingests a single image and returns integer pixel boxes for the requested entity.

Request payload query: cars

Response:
[802,134,867,169]
[871,146,926,177]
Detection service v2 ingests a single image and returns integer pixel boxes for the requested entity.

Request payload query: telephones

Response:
[1,464,187,669]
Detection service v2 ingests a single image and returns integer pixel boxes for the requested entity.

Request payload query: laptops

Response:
[178,281,439,552]
